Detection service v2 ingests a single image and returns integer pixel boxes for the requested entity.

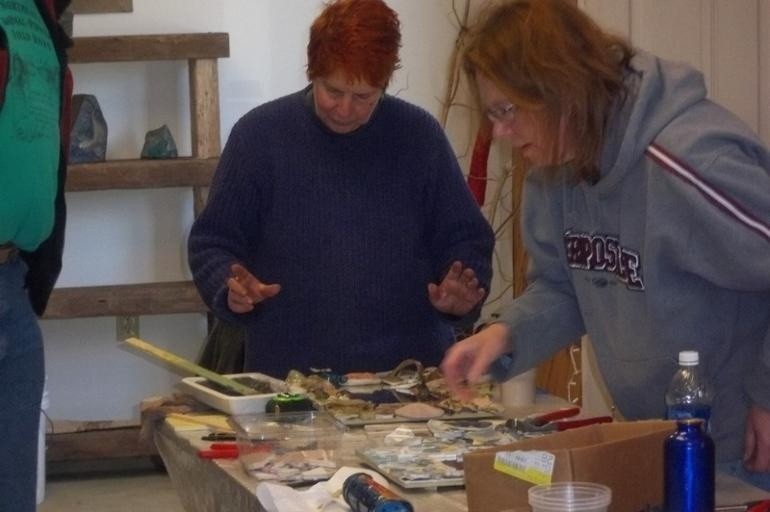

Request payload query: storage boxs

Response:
[463,420,705,512]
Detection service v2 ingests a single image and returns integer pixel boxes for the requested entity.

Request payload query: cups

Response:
[499,368,535,408]
[526,484,613,512]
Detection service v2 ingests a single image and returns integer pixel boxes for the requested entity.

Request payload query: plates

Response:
[180,370,300,418]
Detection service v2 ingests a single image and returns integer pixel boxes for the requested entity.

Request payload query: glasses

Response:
[483,87,538,126]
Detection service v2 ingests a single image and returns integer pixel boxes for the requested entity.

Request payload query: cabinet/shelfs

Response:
[40,0,229,481]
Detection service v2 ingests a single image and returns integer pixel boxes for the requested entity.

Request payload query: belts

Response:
[0,245,21,264]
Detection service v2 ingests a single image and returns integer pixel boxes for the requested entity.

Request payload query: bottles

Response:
[660,419,718,510]
[338,473,411,512]
[665,347,715,429]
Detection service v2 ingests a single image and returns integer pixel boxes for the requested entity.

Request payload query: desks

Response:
[140,386,770,512]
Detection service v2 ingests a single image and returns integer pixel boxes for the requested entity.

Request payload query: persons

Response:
[1,0,75,511]
[184,1,495,380]
[435,3,770,491]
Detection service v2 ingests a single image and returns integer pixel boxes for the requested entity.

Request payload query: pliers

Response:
[198,443,318,457]
[504,407,614,430]
